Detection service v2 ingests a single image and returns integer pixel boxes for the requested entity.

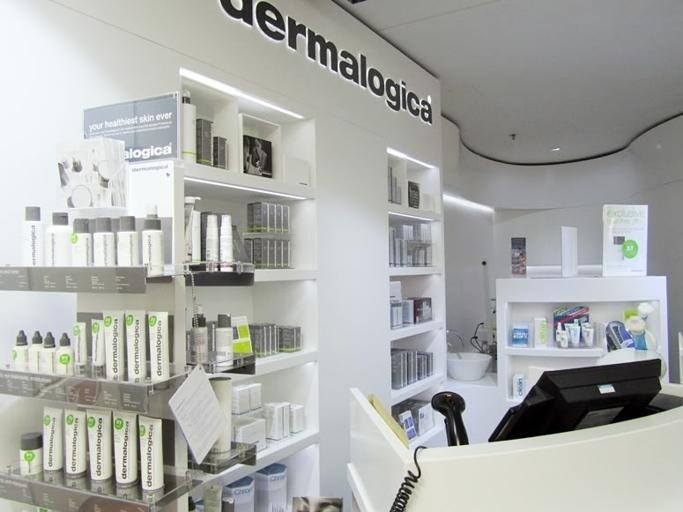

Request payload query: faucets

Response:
[447,329,465,345]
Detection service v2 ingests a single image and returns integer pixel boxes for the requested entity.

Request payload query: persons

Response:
[514,247,526,274]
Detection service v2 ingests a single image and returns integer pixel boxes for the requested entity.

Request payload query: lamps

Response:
[431,390,469,447]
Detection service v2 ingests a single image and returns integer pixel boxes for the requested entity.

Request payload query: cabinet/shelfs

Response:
[0,159,176,512]
[496,274,670,387]
[176,67,322,512]
[386,148,447,450]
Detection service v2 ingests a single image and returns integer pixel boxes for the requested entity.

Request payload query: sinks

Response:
[447,353,493,380]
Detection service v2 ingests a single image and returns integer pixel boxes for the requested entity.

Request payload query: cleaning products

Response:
[22,206,164,278]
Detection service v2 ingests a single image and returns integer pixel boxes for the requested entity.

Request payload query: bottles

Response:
[181,97,197,161]
[555,322,562,342]
[116,215,139,267]
[613,236,625,260]
[92,217,114,266]
[18,431,42,477]
[141,214,165,278]
[204,213,234,273]
[47,211,73,269]
[71,218,92,268]
[22,206,41,266]
[511,372,524,400]
[189,313,234,366]
[12,327,72,377]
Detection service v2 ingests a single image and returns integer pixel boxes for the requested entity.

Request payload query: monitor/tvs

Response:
[487,358,663,442]
